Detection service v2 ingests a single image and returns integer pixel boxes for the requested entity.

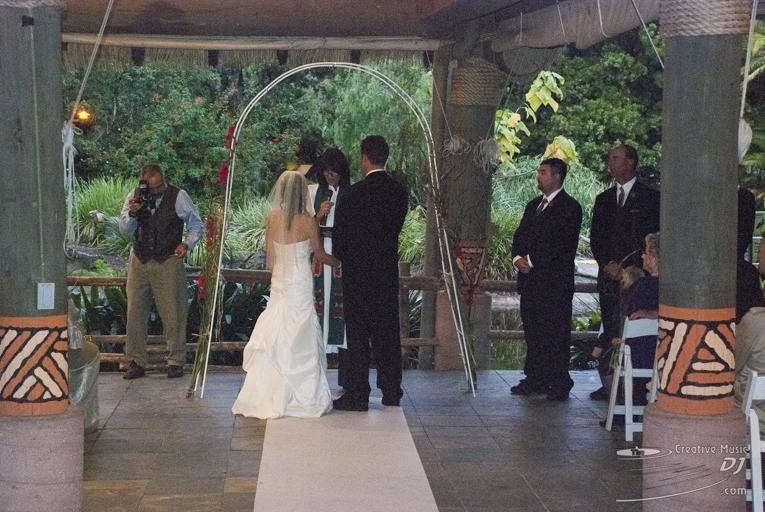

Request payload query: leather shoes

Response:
[510,375,639,425]
[332,393,369,412]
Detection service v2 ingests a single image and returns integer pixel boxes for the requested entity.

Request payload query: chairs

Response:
[735,367,765,512]
[605,317,659,442]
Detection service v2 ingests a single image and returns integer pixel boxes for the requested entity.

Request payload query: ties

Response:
[534,197,548,217]
[615,184,625,206]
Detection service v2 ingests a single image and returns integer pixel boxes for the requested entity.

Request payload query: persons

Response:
[589,143,662,400]
[305,145,352,389]
[506,155,585,402]
[735,183,765,451]
[330,134,409,413]
[594,231,662,430]
[230,169,343,421]
[115,162,207,382]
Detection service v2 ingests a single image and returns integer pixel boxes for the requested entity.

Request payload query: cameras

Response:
[134,180,151,218]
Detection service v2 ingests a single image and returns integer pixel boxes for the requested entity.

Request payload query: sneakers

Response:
[167,363,184,378]
[123,359,145,378]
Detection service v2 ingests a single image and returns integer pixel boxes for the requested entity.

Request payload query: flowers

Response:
[186,122,237,398]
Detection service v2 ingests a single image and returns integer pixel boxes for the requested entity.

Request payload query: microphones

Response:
[325,190,333,220]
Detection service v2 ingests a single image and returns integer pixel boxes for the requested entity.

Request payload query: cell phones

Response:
[170,248,178,257]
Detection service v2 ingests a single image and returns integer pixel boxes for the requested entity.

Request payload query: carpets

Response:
[252,395,440,512]
